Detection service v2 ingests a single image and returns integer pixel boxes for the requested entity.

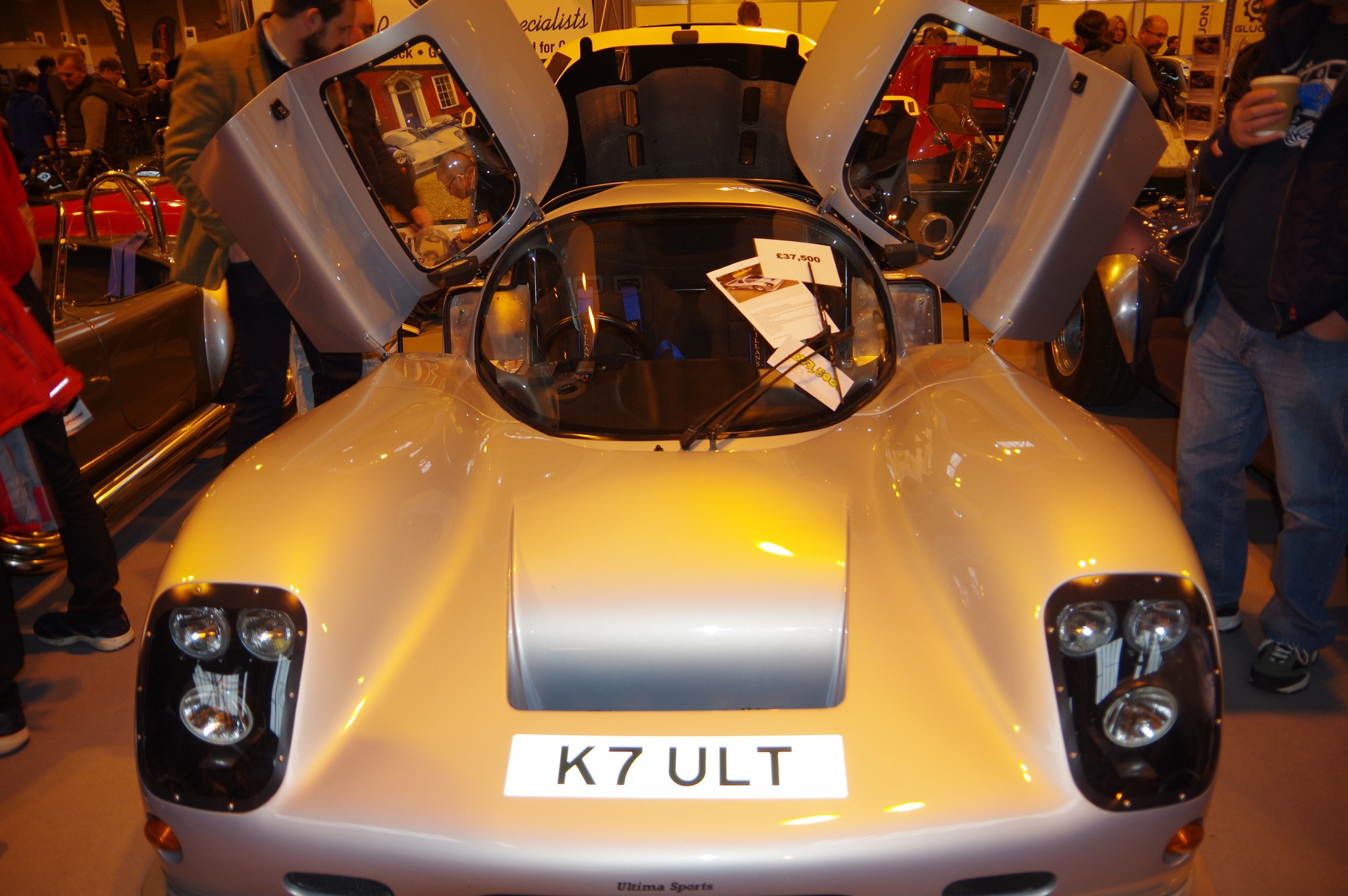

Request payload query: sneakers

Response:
[31,606,133,650]
[1250,638,1318,691]
[0,698,31,754]
[1210,601,1242,631]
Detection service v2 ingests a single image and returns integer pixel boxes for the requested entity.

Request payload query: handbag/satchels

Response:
[1151,96,1191,178]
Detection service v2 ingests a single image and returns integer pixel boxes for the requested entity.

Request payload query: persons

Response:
[1158,0,1348,692]
[436,152,476,200]
[920,13,1186,135]
[164,0,363,470]
[0,47,181,176]
[339,0,432,239]
[0,123,135,755]
[737,0,762,27]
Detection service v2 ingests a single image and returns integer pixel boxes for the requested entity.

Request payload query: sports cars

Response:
[0,0,1223,896]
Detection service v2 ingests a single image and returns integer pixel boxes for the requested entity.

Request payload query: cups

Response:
[1249,74,1300,138]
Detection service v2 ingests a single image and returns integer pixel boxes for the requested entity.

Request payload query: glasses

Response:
[1144,28,1170,43]
[446,173,463,192]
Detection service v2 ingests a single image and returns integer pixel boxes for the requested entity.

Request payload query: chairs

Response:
[704,217,844,358]
[532,223,689,363]
